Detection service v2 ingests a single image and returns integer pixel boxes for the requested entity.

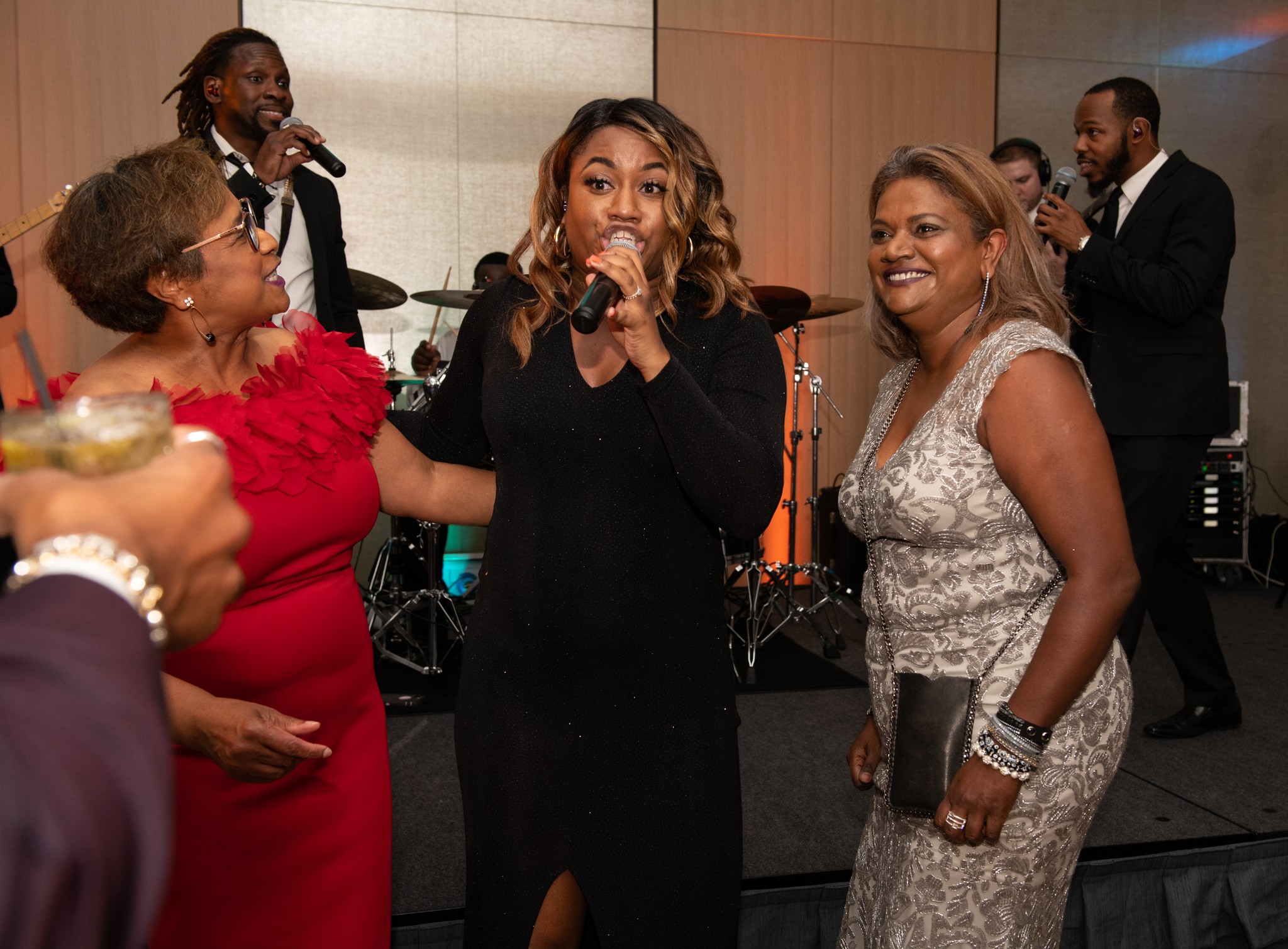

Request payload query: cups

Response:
[0,392,173,476]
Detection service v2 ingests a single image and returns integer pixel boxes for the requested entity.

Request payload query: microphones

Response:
[1036,166,1077,248]
[280,117,346,178]
[571,242,642,335]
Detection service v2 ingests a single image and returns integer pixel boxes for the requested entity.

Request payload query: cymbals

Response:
[385,370,427,386]
[346,268,408,311]
[409,289,485,310]
[748,285,865,335]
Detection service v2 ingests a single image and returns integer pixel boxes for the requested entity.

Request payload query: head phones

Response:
[990,138,1052,186]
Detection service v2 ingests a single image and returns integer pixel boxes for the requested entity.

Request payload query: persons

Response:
[831,141,1145,949]
[42,141,493,949]
[984,132,1055,222]
[384,96,788,949]
[1040,75,1248,747]
[160,25,369,347]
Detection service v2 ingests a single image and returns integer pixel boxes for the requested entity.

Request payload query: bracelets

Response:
[967,700,1055,787]
[0,386,254,949]
[12,536,170,661]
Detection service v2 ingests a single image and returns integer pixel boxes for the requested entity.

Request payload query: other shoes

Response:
[1143,707,1243,744]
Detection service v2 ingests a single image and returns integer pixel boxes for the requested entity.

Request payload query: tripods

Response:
[367,521,469,717]
[722,319,871,688]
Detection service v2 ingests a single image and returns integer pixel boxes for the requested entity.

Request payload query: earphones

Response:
[207,84,219,97]
[1133,125,1142,138]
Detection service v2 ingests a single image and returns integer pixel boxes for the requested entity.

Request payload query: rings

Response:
[946,811,966,830]
[172,432,224,454]
[621,286,642,299]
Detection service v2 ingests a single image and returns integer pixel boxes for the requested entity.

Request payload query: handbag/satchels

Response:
[884,671,980,820]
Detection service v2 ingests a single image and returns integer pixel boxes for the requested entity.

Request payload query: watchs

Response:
[1072,228,1094,255]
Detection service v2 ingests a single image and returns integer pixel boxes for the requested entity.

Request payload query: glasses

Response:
[164,196,260,261]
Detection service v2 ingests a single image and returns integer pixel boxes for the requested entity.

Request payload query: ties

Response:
[1093,186,1123,239]
